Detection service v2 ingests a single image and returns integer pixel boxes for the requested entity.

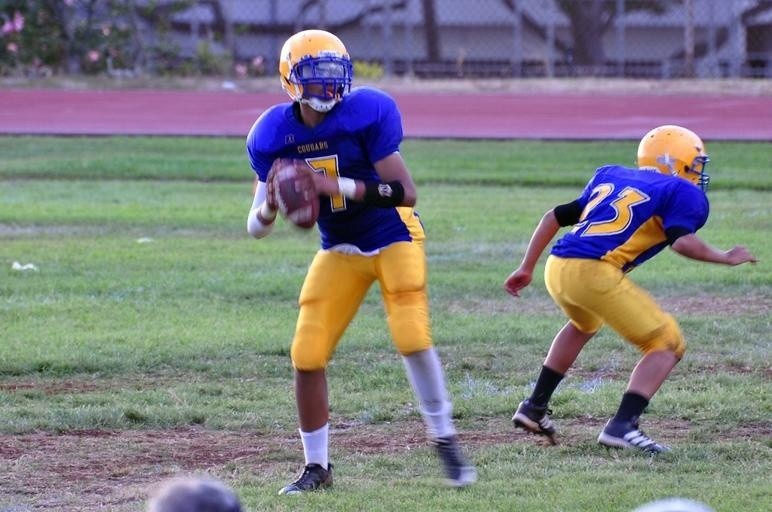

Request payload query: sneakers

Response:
[597,418,669,457]
[512,400,560,445]
[432,434,476,487]
[278,462,334,495]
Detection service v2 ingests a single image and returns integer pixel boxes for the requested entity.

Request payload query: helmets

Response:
[279,29,354,114]
[637,125,709,194]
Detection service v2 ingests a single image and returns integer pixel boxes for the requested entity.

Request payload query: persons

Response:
[507,126,759,456]
[246,30,481,497]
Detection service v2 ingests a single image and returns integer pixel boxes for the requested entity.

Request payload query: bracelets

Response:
[258,202,276,220]
[361,179,405,208]
[338,174,358,199]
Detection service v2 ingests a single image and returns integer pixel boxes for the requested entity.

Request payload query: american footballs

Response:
[271,159,320,228]
